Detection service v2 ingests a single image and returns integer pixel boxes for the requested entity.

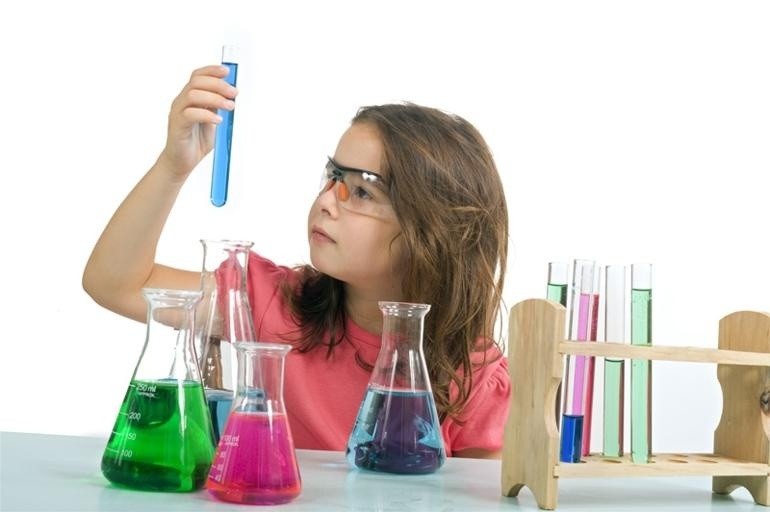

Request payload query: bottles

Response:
[208,45,240,206]
[601,261,624,462]
[96,286,218,492]
[539,260,570,437]
[348,300,446,479]
[629,258,653,465]
[560,261,604,462]
[172,237,265,444]
[208,341,304,509]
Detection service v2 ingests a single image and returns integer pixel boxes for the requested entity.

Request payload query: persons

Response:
[80,65,519,460]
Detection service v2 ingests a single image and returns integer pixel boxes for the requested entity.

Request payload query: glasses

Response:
[319,156,398,218]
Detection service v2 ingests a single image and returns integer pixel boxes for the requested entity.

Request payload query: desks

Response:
[1,430,770,511]
